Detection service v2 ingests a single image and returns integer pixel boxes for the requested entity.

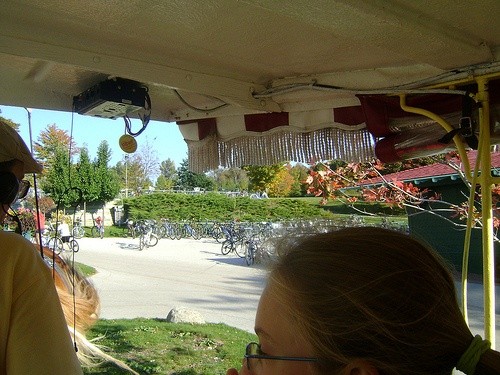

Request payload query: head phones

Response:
[0,170,20,204]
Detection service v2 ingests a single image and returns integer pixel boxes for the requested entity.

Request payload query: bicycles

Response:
[71,216,85,239]
[138,222,158,251]
[43,228,63,255]
[91,216,105,239]
[55,228,79,253]
[123,214,408,267]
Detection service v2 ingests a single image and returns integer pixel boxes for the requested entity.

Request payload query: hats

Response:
[0,121,41,173]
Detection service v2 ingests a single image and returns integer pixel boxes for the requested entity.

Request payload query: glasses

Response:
[244,343,343,370]
[17,179,32,199]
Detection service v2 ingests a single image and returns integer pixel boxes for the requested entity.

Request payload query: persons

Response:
[0,120,85,375]
[57,219,72,251]
[35,211,45,244]
[96,216,104,239]
[226,225,500,375]
